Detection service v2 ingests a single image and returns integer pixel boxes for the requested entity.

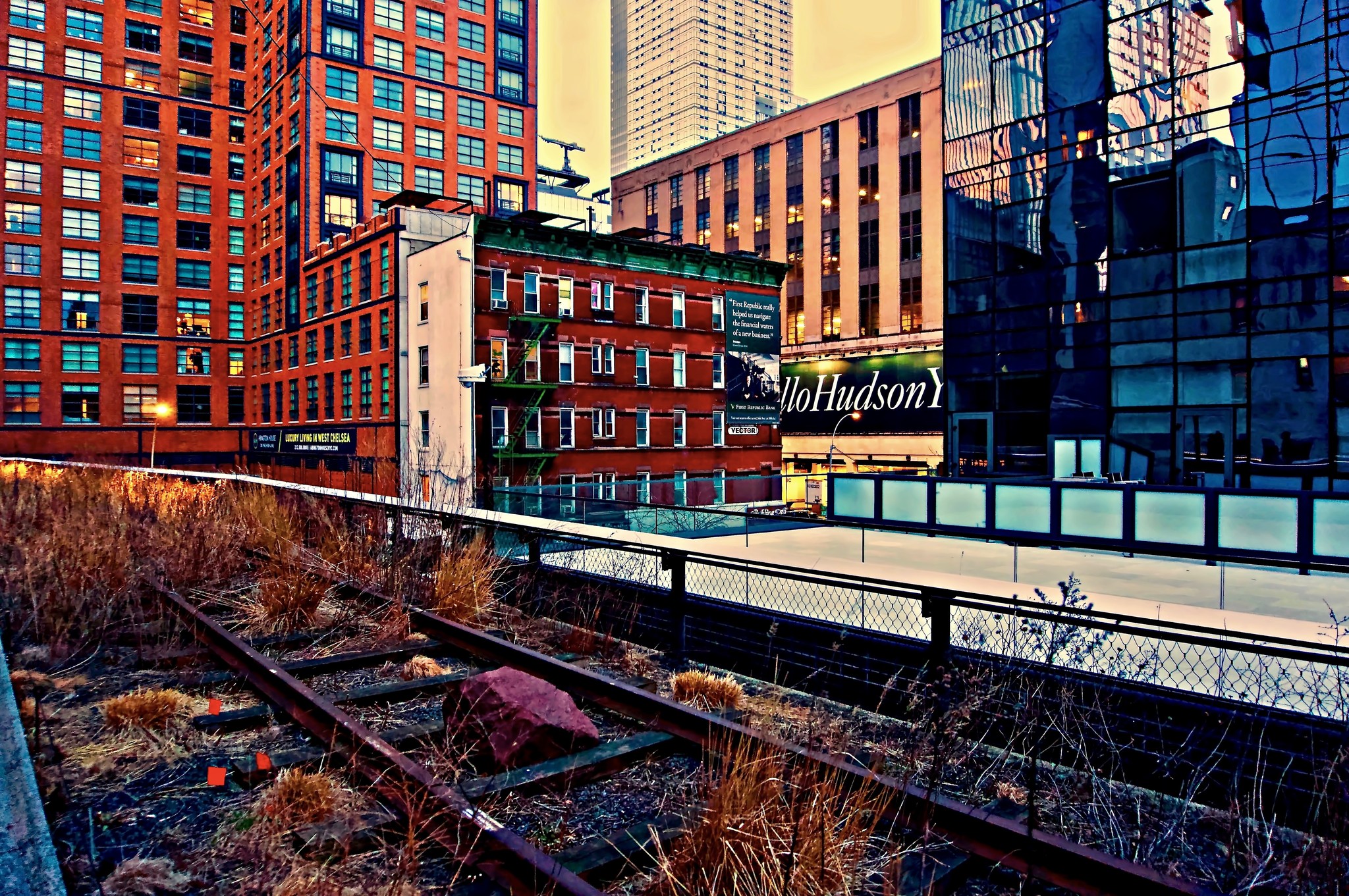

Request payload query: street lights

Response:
[829,413,861,473]
[150,403,174,469]
